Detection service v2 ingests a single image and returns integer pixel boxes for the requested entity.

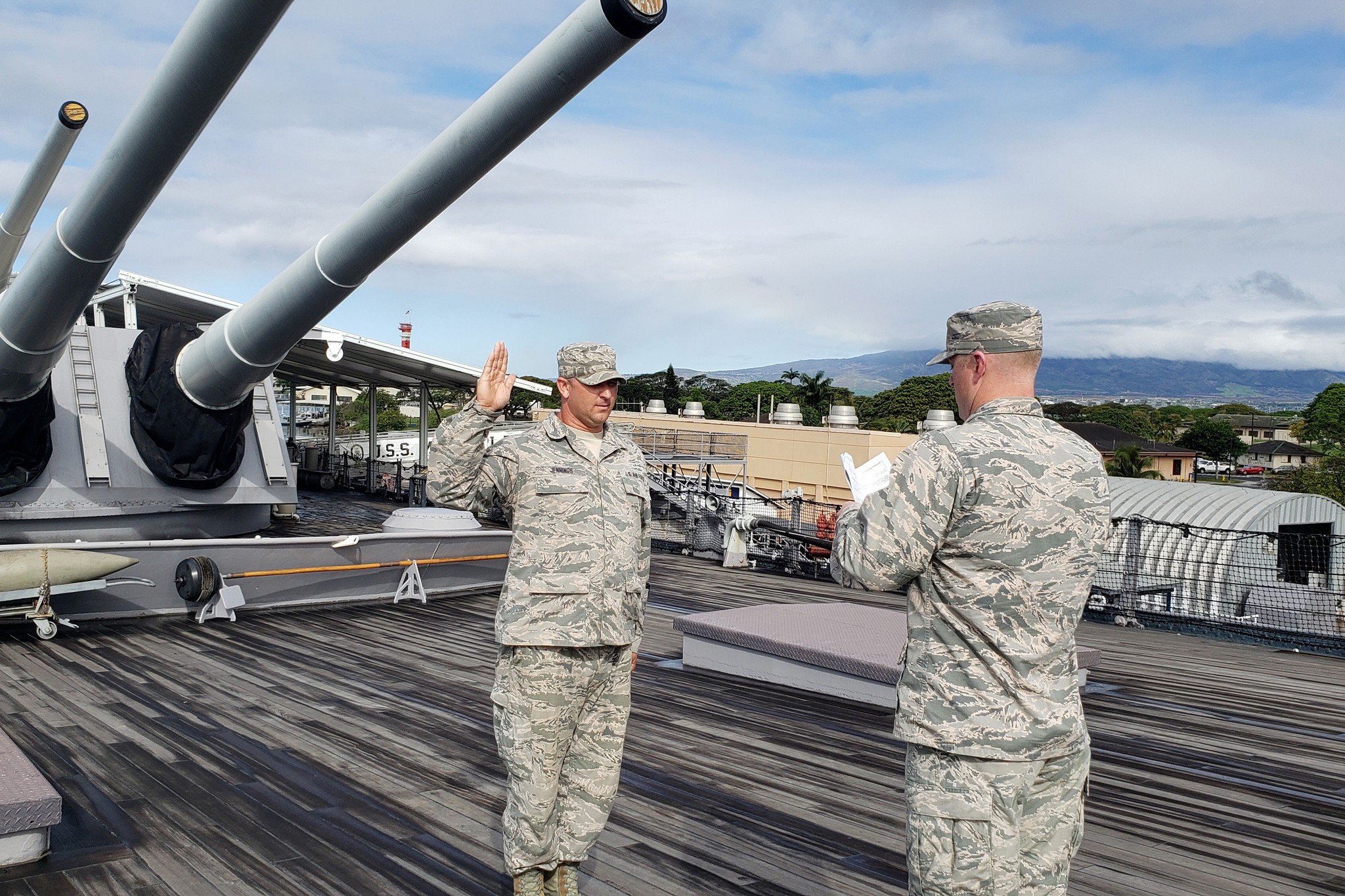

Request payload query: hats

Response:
[926,300,1043,366]
[557,342,627,385]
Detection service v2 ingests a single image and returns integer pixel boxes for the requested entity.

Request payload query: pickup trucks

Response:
[1197,461,1235,475]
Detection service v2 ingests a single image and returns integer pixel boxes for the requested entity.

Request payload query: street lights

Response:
[1248,412,1254,445]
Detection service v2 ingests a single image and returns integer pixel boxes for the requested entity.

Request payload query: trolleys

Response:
[0,576,157,640]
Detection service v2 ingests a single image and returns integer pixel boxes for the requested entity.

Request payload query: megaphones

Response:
[705,495,719,511]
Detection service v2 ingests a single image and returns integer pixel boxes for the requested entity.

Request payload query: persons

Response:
[380,473,393,491]
[426,341,652,896]
[286,436,297,462]
[830,302,1114,896]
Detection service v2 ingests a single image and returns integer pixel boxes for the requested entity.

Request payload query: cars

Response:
[1270,465,1300,476]
[1236,465,1264,475]
[1193,457,1208,470]
[1219,462,1233,466]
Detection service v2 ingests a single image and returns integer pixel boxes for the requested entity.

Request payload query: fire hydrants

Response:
[1222,476,1226,481]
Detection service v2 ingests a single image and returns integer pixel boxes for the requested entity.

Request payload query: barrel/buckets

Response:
[1189,473,1195,481]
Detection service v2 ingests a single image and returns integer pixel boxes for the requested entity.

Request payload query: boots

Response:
[543,862,581,896]
[514,867,545,896]
